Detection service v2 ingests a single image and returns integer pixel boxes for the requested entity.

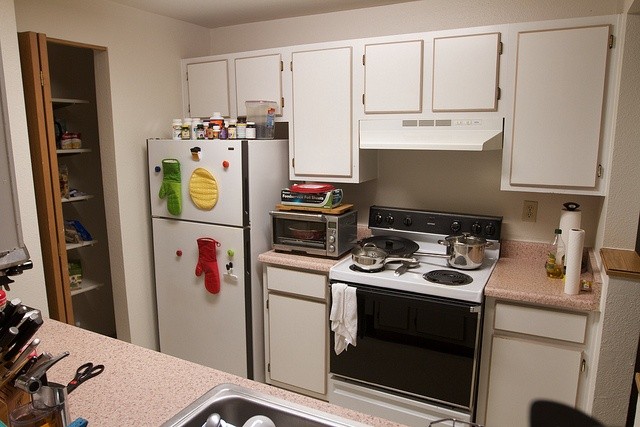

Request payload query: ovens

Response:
[331,281,481,411]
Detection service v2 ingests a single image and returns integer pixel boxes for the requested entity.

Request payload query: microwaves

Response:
[269,209,358,258]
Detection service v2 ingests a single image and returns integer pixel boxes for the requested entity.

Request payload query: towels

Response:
[331,283,357,355]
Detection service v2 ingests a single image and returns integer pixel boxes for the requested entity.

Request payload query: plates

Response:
[292,183,334,193]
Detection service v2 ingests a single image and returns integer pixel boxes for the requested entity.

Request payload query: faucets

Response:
[205,411,222,427]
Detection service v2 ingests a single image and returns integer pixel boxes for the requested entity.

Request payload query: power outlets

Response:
[522,200,538,222]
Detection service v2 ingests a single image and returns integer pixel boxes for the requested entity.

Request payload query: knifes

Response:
[0,297,43,395]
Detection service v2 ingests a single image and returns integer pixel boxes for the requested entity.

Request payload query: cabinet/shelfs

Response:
[288,38,376,183]
[180,48,288,124]
[500,13,622,197]
[53,98,105,297]
[264,266,328,403]
[360,18,504,121]
[477,298,600,427]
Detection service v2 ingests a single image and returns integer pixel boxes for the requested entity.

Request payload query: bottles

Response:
[228,122,237,139]
[172,119,182,138]
[545,230,566,280]
[72,134,81,149]
[184,118,193,125]
[213,126,220,138]
[265,110,275,139]
[192,118,202,140]
[219,123,227,139]
[196,125,204,139]
[203,120,210,139]
[210,112,224,126]
[61,135,72,149]
[246,121,256,139]
[208,126,214,139]
[237,116,246,138]
[181,125,190,140]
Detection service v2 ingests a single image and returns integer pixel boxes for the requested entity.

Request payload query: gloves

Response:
[159,158,182,215]
[196,237,221,294]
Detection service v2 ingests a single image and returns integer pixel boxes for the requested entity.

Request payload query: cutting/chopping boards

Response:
[275,201,352,214]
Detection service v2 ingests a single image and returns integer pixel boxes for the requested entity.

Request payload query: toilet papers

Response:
[565,228,586,295]
[555,209,581,265]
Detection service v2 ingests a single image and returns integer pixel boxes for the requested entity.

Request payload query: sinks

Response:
[160,383,333,427]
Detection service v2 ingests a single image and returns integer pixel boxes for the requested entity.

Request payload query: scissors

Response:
[67,362,104,394]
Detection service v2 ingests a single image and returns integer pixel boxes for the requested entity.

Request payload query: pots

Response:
[438,231,492,271]
[352,243,418,270]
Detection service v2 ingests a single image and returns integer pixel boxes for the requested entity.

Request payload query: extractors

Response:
[359,118,504,152]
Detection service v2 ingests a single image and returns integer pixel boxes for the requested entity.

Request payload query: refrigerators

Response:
[146,139,290,383]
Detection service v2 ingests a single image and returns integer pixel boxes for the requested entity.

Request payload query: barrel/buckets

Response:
[245,101,277,140]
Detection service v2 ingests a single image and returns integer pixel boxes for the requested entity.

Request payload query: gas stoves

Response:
[328,241,501,291]
